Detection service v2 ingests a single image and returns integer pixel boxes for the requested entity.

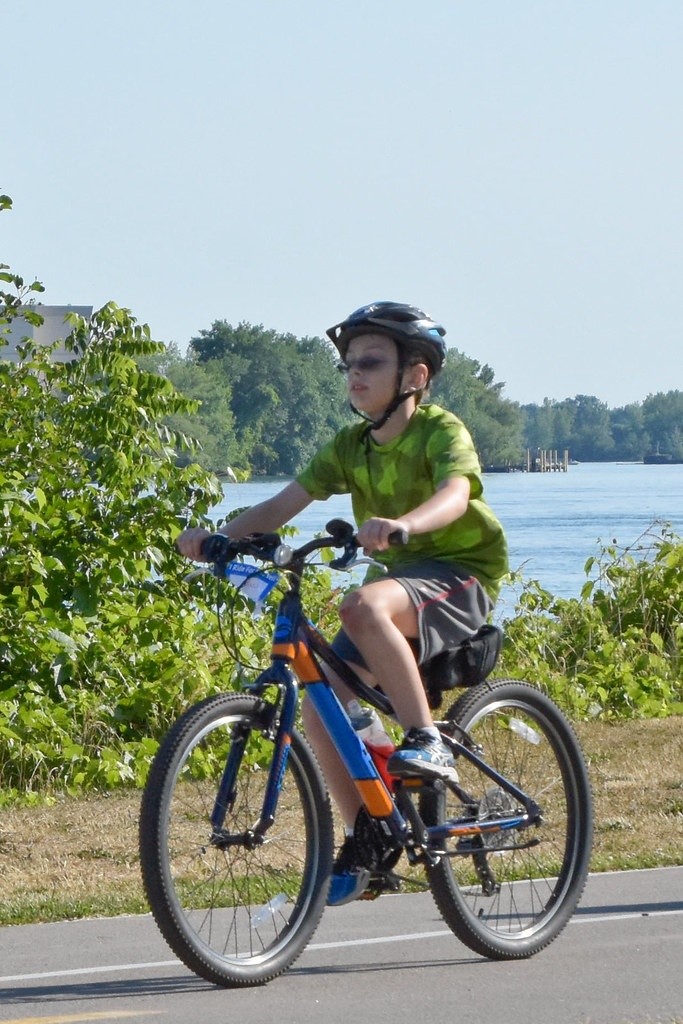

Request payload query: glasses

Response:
[337,356,411,374]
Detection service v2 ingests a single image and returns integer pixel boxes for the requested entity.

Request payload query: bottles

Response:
[347,699,401,793]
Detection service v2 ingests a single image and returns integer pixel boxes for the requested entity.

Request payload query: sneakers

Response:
[326,834,393,907]
[387,727,460,784]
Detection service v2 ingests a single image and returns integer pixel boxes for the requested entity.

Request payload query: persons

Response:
[176,301,509,907]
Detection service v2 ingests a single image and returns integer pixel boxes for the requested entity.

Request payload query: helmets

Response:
[325,301,445,376]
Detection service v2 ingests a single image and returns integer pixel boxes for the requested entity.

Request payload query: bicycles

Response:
[123,523,597,985]
